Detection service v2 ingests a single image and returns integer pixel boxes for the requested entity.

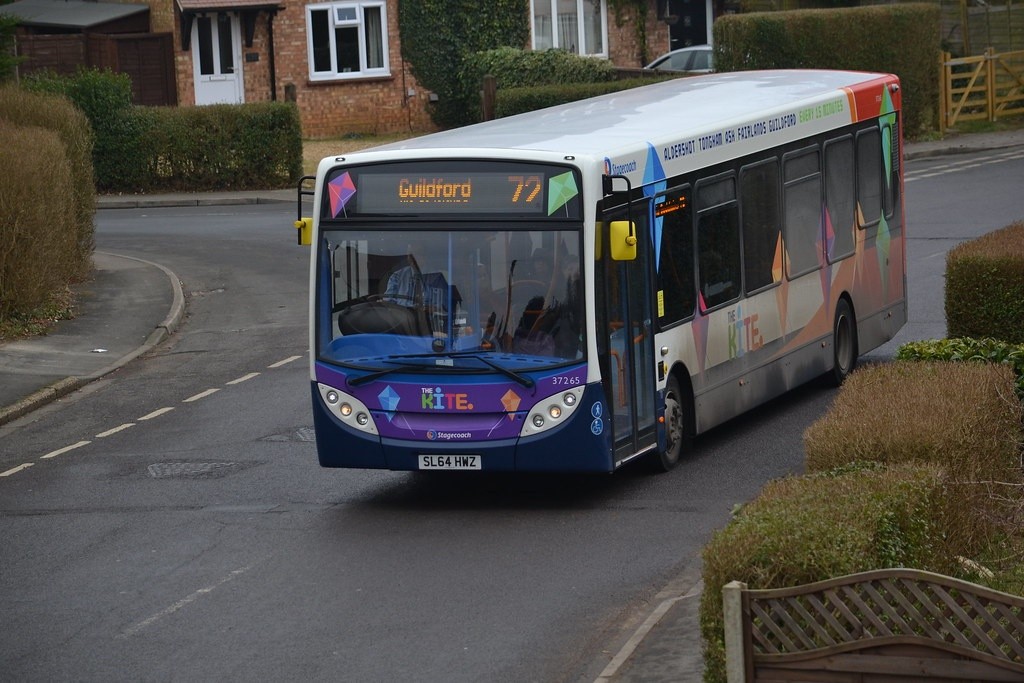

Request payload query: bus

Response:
[297,66,911,477]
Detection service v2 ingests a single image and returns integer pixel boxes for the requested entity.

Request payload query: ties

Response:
[413,279,423,305]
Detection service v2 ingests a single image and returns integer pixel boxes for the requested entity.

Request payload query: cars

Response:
[642,45,715,73]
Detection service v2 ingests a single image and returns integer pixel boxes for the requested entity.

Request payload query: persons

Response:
[375,242,448,333]
